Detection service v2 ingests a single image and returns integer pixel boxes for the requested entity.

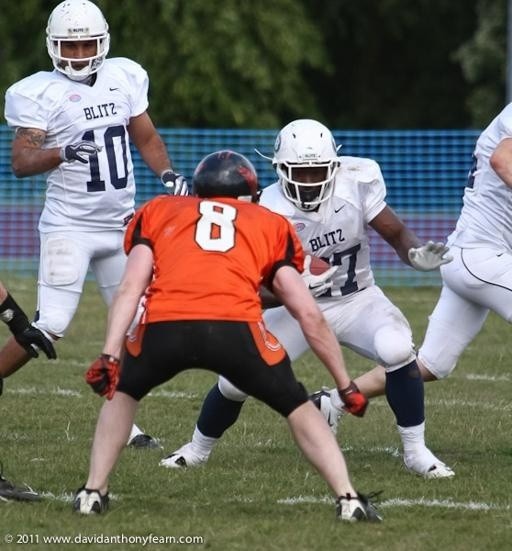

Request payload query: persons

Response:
[159,116,457,482]
[310,96,510,438]
[0,282,56,504]
[0,3,187,444]
[72,151,383,525]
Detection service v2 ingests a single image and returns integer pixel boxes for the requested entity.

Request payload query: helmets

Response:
[45,0,111,79]
[193,149,258,208]
[270,118,340,212]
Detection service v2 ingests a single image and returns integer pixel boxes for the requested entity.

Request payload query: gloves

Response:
[336,381,367,416]
[13,323,61,362]
[408,239,452,271]
[161,170,190,199]
[63,139,102,165]
[85,355,120,400]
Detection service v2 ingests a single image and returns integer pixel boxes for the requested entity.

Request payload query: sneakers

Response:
[125,423,156,452]
[157,438,212,471]
[72,485,112,517]
[305,386,341,435]
[334,492,384,525]
[402,447,457,480]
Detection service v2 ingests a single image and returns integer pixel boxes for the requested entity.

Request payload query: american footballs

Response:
[303,251,330,275]
